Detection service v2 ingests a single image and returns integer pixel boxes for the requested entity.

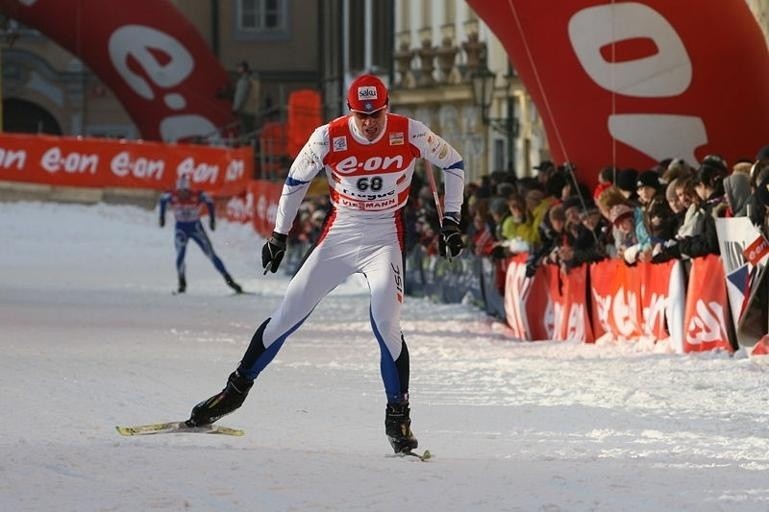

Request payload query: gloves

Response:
[261,231,288,274]
[159,215,165,226]
[438,211,464,259]
[209,219,216,230]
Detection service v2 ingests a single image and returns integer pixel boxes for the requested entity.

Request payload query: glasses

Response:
[351,106,386,119]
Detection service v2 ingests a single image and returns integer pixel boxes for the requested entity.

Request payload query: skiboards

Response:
[114,420,431,463]
[171,290,259,297]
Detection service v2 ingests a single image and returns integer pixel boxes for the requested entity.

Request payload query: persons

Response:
[285,195,331,275]
[158,169,244,296]
[403,172,445,249]
[458,144,769,277]
[190,73,466,449]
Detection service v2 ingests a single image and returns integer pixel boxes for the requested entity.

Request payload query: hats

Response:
[592,155,755,227]
[175,179,191,192]
[470,161,599,223]
[347,75,389,114]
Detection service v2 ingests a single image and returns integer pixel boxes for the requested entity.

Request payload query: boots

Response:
[178,277,185,292]
[383,401,418,448]
[191,371,254,425]
[223,273,242,292]
